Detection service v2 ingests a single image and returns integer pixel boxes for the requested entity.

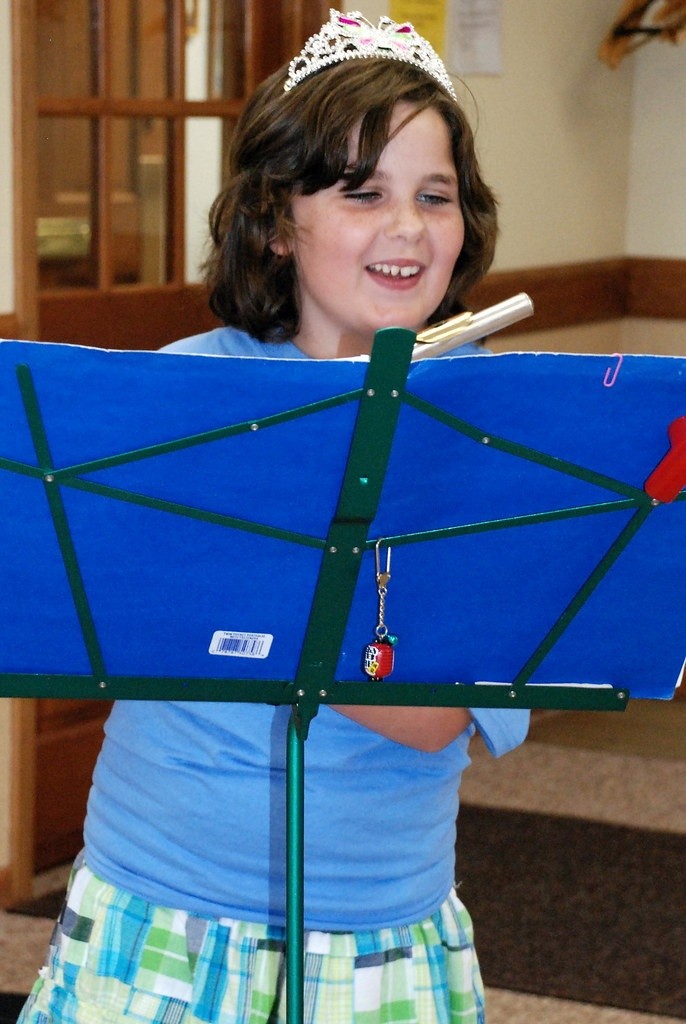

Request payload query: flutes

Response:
[351,286,535,362]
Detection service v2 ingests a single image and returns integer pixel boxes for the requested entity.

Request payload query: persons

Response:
[19,7,529,1022]
